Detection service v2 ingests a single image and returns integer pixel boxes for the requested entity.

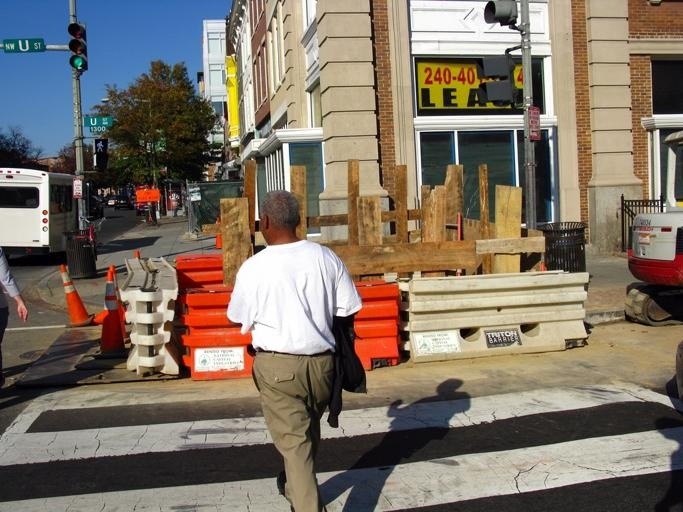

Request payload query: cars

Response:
[91,195,134,220]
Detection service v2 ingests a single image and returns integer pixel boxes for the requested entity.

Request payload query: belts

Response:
[257,348,335,358]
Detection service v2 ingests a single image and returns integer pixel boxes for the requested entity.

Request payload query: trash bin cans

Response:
[61,230,96,279]
[536,222,588,273]
[145,207,156,225]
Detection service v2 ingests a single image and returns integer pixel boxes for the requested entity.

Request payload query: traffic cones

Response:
[60,264,131,359]
[216,217,222,249]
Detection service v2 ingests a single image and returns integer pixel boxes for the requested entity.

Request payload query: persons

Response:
[222,191,364,512]
[0,247,26,390]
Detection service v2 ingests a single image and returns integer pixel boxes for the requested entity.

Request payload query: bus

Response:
[0,167,77,259]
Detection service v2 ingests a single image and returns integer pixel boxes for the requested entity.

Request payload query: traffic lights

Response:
[95,138,108,169]
[476,0,518,104]
[68,23,88,73]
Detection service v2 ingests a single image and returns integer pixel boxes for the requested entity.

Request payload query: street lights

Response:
[101,97,156,185]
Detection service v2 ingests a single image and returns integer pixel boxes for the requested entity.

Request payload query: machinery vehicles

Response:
[625,131,683,326]
